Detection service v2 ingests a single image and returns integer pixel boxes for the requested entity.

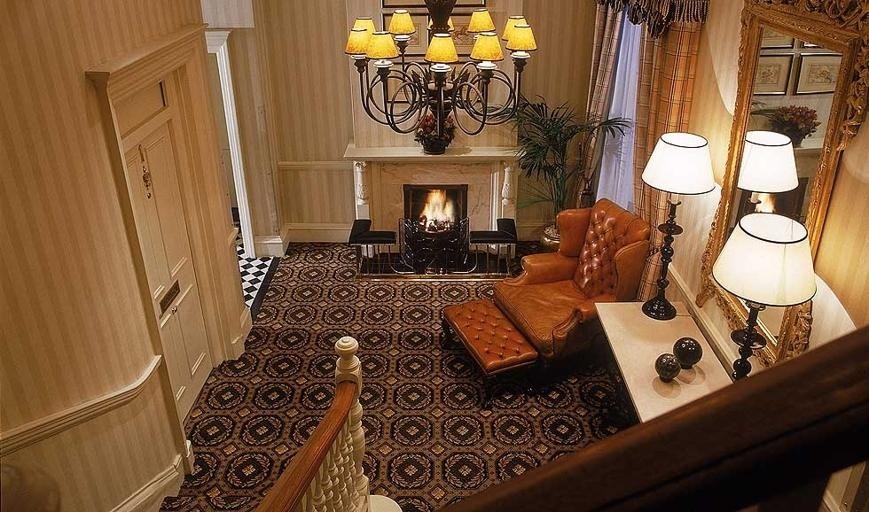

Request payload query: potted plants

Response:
[480,89,634,255]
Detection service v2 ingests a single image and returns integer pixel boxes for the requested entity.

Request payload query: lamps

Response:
[713,213,817,380]
[640,131,716,321]
[737,130,800,211]
[345,0,538,139]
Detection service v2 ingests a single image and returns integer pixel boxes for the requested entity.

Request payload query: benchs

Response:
[439,299,540,402]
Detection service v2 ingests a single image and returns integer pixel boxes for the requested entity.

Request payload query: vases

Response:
[792,141,802,153]
[423,143,448,156]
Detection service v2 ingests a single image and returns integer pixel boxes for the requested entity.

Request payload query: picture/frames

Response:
[755,54,795,99]
[380,11,429,57]
[446,62,481,101]
[449,12,479,57]
[760,23,794,51]
[453,0,486,7]
[381,1,428,9]
[796,52,843,96]
[384,61,430,103]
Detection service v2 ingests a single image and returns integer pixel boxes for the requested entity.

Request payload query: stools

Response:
[470,231,515,245]
[350,219,371,241]
[497,218,516,240]
[352,231,404,246]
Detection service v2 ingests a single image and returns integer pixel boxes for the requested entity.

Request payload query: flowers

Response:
[414,115,456,147]
[767,103,820,146]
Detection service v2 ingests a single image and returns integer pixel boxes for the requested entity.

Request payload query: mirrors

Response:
[696,0,869,369]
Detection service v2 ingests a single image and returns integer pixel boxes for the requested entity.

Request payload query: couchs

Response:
[493,198,652,381]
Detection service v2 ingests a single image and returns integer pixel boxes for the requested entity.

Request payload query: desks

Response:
[594,300,735,424]
